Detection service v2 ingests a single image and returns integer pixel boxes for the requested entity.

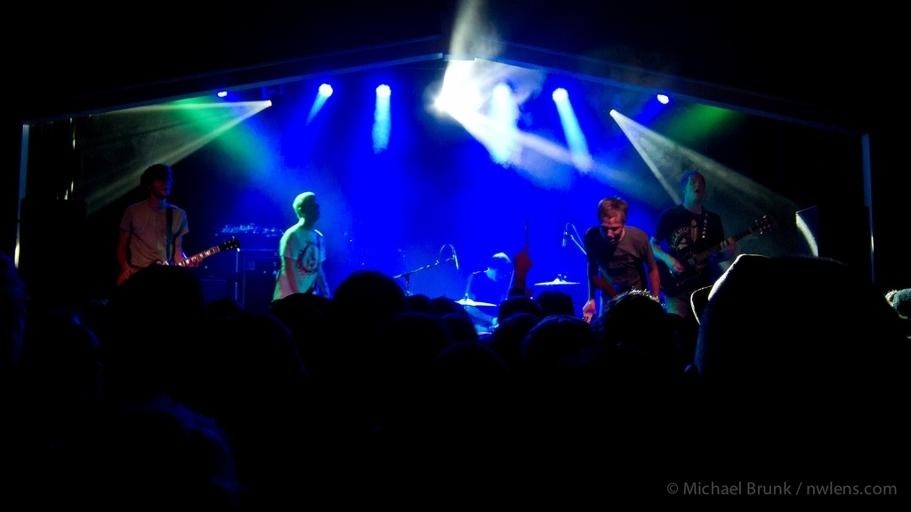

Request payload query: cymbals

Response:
[534,278,581,286]
[455,298,497,307]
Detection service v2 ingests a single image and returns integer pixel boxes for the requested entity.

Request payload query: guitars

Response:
[118,236,237,286]
[656,215,771,297]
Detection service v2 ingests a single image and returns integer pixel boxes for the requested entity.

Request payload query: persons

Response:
[582,197,661,324]
[272,192,330,302]
[115,164,200,285]
[649,171,736,318]
[2,255,911,511]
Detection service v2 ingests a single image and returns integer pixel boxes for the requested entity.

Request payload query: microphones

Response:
[451,254,459,270]
[562,223,568,246]
